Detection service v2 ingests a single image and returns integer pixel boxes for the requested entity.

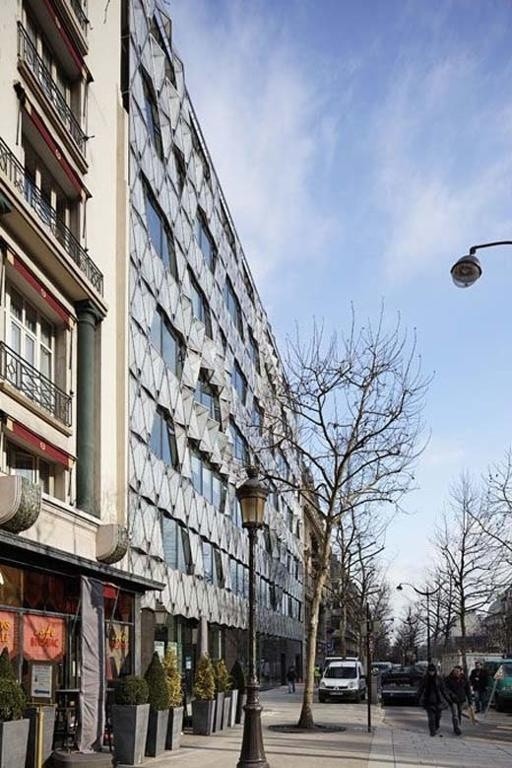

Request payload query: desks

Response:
[52,685,115,754]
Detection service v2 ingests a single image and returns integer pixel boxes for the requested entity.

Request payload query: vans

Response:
[318,660,366,704]
[478,658,511,712]
[319,656,359,674]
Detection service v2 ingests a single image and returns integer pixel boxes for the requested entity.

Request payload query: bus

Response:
[370,660,395,675]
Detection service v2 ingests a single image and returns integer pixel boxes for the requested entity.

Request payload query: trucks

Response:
[436,649,507,704]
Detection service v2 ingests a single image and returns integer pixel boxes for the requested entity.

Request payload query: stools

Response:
[107,644,249,765]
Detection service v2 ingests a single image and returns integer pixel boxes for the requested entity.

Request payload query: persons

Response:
[287,667,297,693]
[443,665,470,735]
[415,663,443,735]
[314,664,321,687]
[319,663,324,678]
[469,661,489,713]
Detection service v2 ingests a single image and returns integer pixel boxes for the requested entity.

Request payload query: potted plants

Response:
[0,646,34,767]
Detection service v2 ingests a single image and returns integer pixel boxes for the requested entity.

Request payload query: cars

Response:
[243,654,282,680]
[377,665,429,705]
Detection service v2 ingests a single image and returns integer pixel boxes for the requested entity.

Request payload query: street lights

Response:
[450,240,512,290]
[395,577,461,669]
[226,463,273,767]
[388,614,429,648]
